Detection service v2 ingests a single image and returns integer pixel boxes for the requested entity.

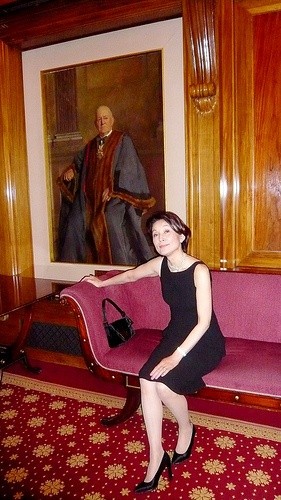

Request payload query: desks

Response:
[0,274,65,386]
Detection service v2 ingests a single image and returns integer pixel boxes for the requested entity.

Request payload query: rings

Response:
[163,367,167,371]
[90,274,93,277]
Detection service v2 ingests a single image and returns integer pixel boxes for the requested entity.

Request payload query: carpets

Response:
[0,371,281,500]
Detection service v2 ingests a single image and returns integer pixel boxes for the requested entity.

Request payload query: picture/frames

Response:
[21,16,187,282]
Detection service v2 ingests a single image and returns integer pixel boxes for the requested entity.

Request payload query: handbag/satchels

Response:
[102,298,135,348]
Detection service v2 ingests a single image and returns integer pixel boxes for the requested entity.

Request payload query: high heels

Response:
[135,452,172,492]
[171,424,195,465]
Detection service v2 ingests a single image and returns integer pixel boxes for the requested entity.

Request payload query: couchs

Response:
[59,270,281,427]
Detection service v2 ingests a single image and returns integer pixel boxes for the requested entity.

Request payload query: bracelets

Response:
[177,346,186,357]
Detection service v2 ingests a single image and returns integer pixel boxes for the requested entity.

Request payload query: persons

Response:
[79,212,226,492]
[56,105,154,266]
